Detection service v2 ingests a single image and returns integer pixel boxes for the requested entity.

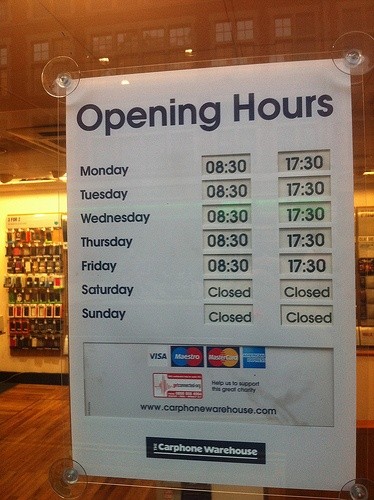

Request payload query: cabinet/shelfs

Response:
[354,205,374,350]
[3,212,69,358]
